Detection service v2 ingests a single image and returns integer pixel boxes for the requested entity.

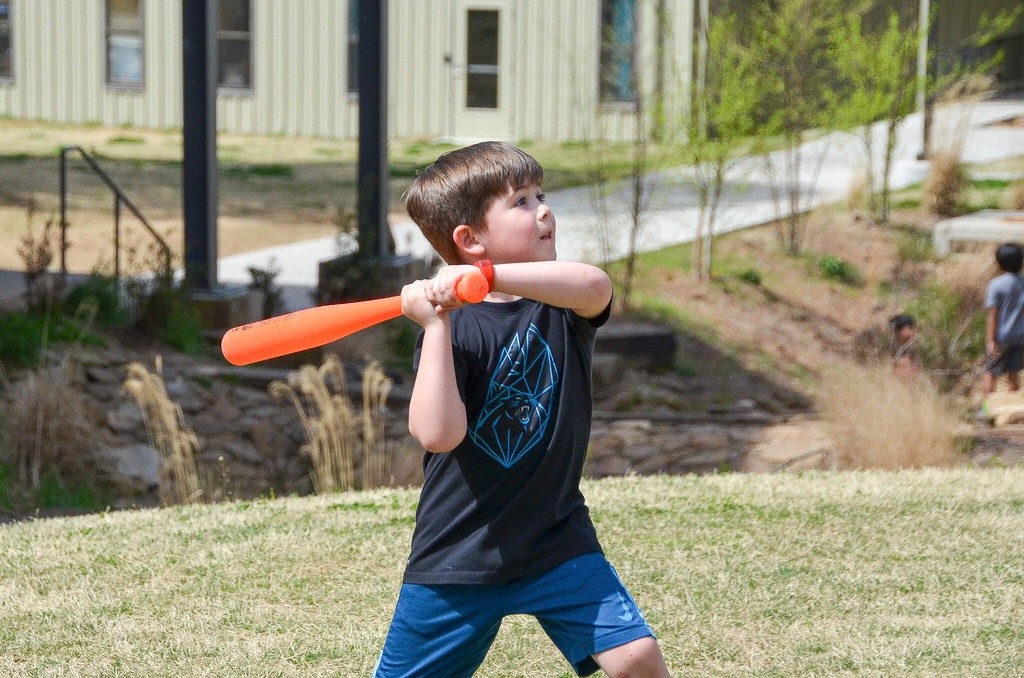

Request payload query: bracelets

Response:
[473,259,494,293]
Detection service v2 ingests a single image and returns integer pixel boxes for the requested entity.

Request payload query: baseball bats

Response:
[220,270,490,368]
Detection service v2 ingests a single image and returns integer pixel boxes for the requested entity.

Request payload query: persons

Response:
[978,242,1024,419]
[889,313,920,382]
[374,140,672,678]
